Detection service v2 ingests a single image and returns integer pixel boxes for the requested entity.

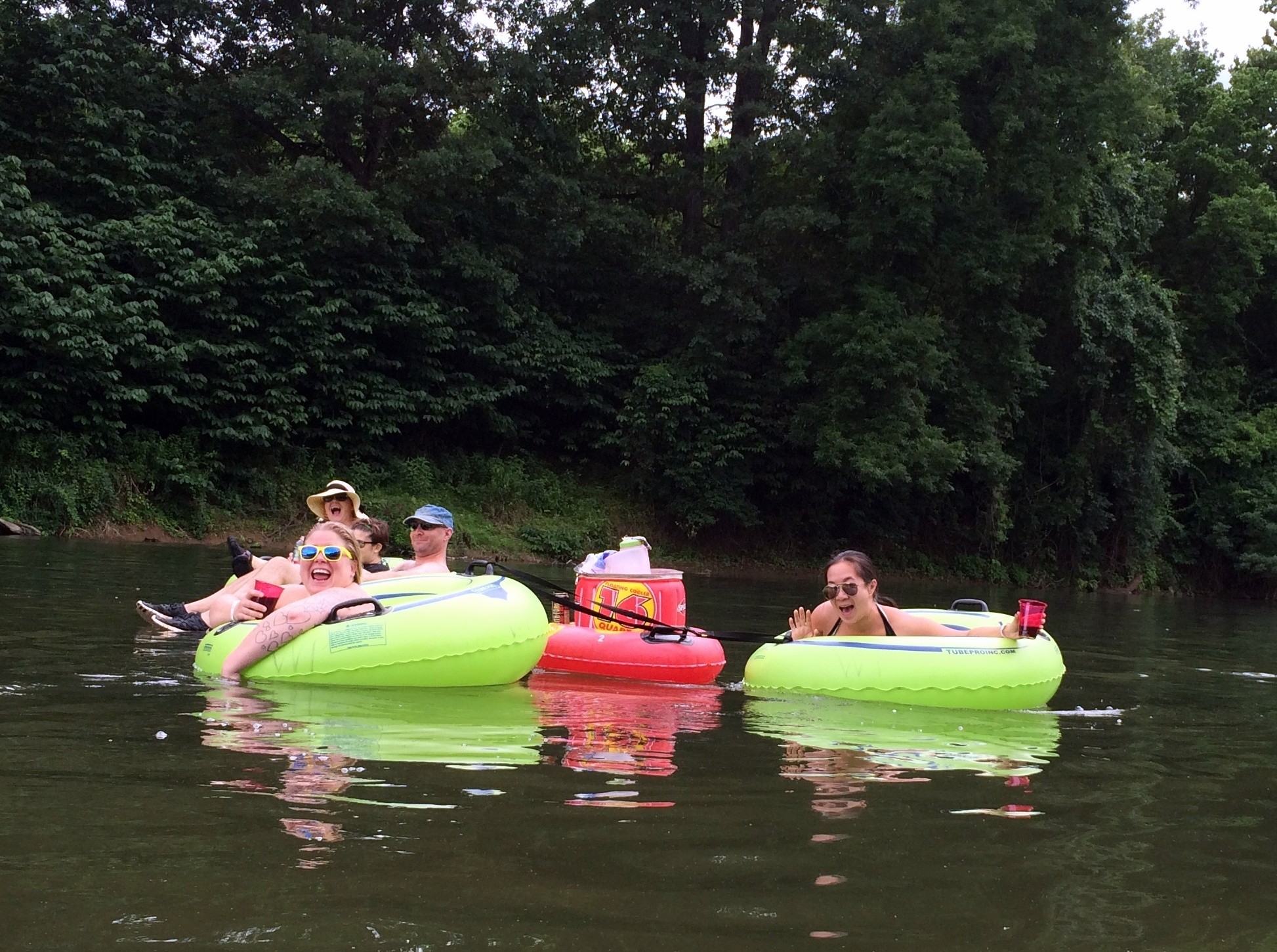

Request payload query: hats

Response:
[403,504,453,530]
[323,492,351,502]
[306,480,369,520]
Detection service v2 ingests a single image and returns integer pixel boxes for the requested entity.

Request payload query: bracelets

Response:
[230,600,240,622]
[1000,625,1007,638]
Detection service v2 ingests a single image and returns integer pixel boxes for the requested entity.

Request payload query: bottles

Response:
[291,536,305,564]
[620,540,644,551]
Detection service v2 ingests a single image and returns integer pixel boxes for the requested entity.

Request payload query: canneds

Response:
[552,592,569,624]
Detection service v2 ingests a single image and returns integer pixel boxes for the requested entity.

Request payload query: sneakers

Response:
[135,600,188,623]
[150,612,213,632]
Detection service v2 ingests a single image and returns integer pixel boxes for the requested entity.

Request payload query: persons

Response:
[789,549,1046,641]
[136,479,455,680]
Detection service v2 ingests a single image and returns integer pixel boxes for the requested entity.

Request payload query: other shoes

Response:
[232,554,254,578]
[227,536,252,563]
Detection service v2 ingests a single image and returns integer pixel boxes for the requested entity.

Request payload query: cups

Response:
[1018,599,1048,640]
[251,580,285,620]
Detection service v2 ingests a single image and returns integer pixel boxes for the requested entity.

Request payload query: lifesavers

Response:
[534,617,726,684]
[223,555,409,587]
[743,604,1067,708]
[193,570,550,689]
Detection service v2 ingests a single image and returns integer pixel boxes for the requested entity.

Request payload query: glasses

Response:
[298,545,353,561]
[821,582,869,600]
[407,522,447,531]
[357,540,376,548]
